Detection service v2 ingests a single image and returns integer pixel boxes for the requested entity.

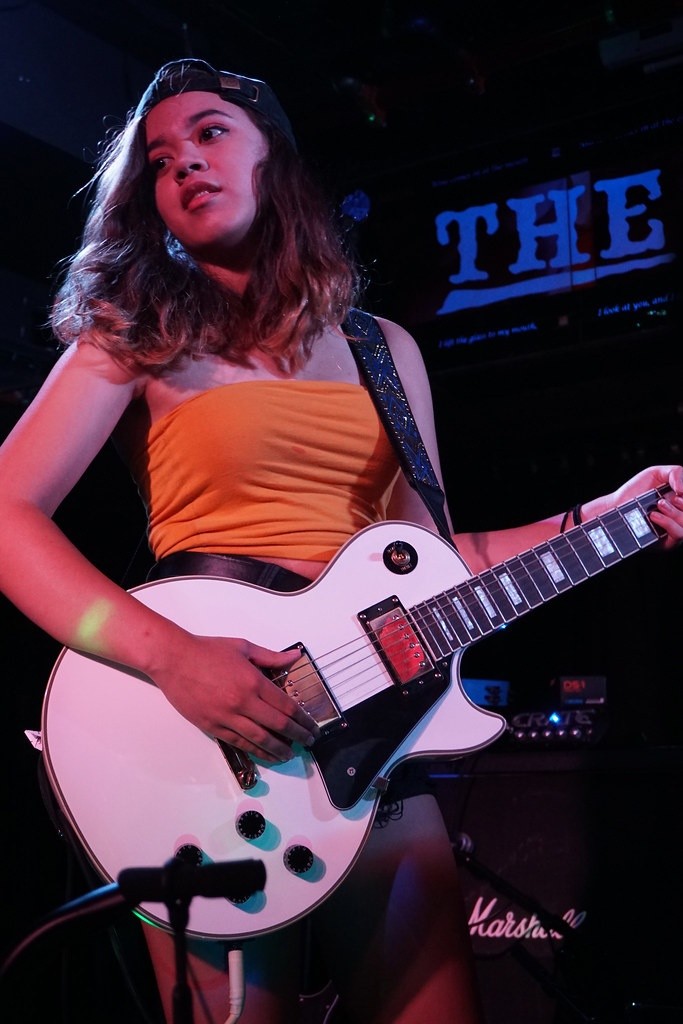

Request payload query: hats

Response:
[138,59,296,157]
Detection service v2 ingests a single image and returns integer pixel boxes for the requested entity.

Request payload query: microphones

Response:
[449,831,474,857]
[119,859,267,902]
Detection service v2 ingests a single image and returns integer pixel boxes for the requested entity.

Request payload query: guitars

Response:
[36,476,675,951]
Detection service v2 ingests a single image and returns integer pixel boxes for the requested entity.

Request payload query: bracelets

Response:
[560,503,582,534]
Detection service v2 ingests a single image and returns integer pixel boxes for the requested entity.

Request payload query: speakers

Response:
[423,749,683,1024]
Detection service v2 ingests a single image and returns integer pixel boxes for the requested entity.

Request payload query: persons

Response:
[0,59,683,1024]
